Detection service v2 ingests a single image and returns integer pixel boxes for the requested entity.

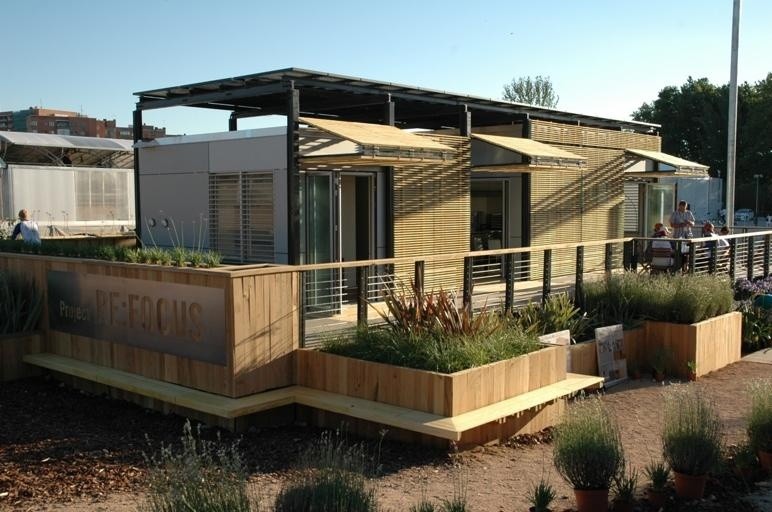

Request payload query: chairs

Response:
[486,239,502,274]
[650,248,683,275]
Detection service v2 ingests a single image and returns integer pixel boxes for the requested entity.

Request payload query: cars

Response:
[735,208,754,222]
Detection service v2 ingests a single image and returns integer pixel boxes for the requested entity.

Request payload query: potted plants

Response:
[0,267,47,382]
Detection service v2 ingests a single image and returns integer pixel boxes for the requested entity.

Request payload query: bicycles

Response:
[719,215,738,226]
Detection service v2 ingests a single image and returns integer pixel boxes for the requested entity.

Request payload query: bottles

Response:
[688,233,693,239]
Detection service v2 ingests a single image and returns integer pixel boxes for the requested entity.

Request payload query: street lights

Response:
[754,174,763,226]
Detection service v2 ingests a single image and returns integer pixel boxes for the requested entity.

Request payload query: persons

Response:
[719,226,729,235]
[645,224,670,262]
[10,207,42,251]
[695,222,730,269]
[667,200,696,272]
